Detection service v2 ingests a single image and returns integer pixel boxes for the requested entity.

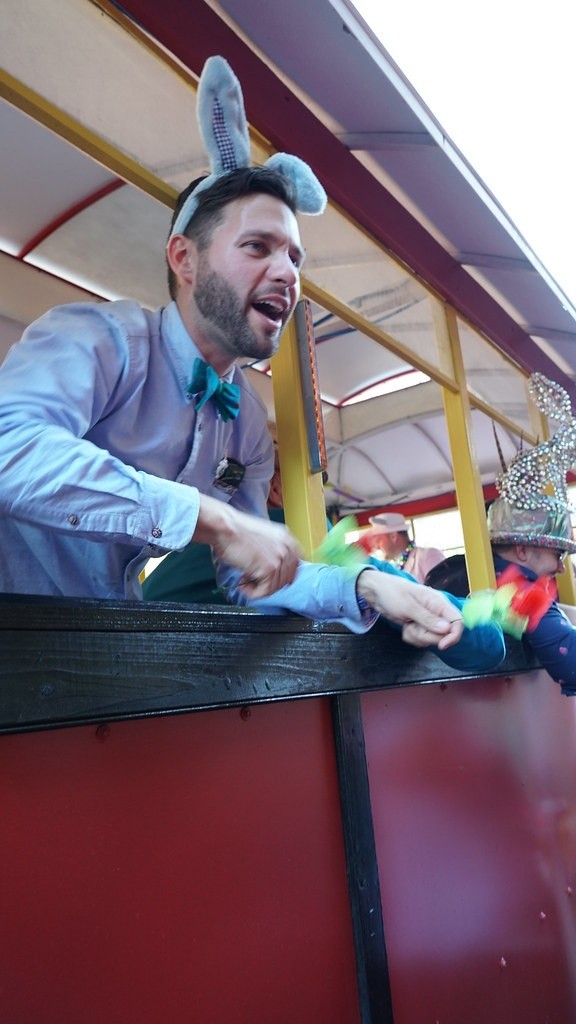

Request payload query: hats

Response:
[368,513,410,535]
[487,372,576,554]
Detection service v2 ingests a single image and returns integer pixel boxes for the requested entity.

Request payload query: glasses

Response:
[547,549,568,564]
[213,456,246,495]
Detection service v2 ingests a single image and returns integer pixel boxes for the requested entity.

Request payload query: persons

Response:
[367,512,446,585]
[0,55,464,648]
[488,494,576,696]
[266,422,506,672]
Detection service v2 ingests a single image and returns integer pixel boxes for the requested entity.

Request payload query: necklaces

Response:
[398,542,414,570]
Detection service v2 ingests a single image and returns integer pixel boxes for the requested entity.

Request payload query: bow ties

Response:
[183,358,241,423]
